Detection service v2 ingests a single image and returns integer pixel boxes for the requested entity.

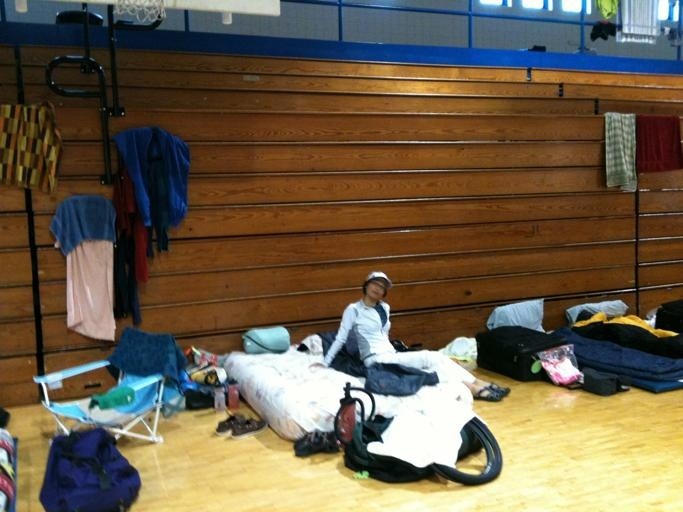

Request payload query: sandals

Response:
[474,382,510,401]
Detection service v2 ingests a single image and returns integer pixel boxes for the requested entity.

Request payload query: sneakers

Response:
[215,414,267,440]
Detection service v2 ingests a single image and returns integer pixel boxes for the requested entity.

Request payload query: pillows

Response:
[241,326,290,353]
[487,297,546,331]
[565,298,629,325]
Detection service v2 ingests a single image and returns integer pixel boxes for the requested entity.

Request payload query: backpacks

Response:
[39,429,140,511]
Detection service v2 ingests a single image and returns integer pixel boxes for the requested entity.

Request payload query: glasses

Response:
[370,281,386,290]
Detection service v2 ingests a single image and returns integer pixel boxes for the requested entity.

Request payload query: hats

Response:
[366,272,393,291]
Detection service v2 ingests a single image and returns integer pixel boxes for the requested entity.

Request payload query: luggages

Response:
[475,325,567,382]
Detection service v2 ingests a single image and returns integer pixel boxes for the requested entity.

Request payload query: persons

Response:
[308,270,511,401]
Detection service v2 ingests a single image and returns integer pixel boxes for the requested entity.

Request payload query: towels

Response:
[117,128,191,280]
[88,389,135,413]
[107,326,187,398]
[2,104,63,192]
[50,195,119,341]
[603,111,683,194]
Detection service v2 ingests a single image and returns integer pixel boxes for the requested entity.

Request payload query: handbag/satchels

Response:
[583,369,620,397]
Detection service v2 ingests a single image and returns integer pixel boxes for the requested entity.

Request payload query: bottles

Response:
[214,387,225,410]
[228,384,240,409]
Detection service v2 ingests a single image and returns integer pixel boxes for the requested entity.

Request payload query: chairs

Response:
[33,325,173,445]
